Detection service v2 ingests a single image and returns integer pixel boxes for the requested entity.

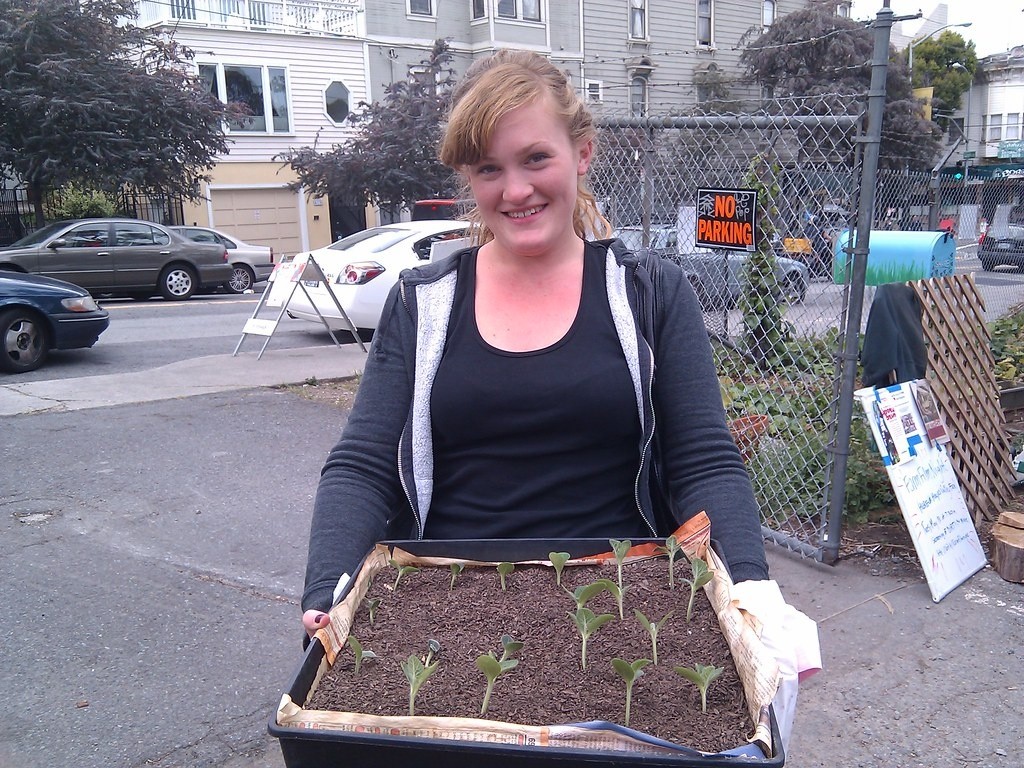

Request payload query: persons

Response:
[302,50,769,695]
[799,214,832,271]
[978,214,989,244]
[880,202,923,231]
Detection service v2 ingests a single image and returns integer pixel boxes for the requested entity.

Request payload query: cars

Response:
[976,203,1024,272]
[0,270,110,375]
[0,218,235,302]
[128,225,275,293]
[612,226,809,309]
[285,219,493,330]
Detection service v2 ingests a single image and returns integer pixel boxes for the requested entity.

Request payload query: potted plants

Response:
[726,400,770,462]
[268,537,786,768]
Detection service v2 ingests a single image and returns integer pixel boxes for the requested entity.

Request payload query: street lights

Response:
[951,62,973,187]
[903,21,973,174]
